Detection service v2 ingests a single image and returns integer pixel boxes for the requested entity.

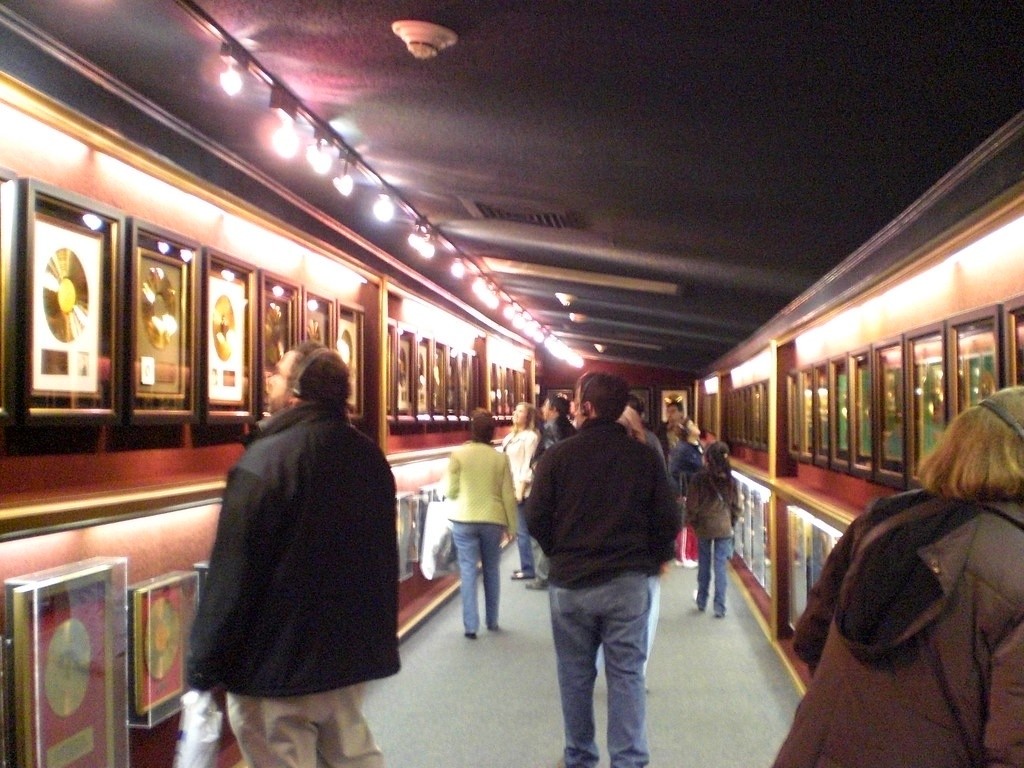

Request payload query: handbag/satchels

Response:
[174,693,226,768]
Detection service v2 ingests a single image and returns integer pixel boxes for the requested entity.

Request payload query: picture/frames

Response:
[0,167,1024,768]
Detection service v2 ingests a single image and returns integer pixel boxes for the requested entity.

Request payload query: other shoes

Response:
[511,569,534,580]
[715,612,725,618]
[696,597,706,612]
[464,633,477,640]
[675,559,698,570]
[526,577,547,590]
[487,621,499,629]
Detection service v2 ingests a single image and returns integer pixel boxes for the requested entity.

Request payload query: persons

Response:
[184,342,402,768]
[442,394,742,638]
[770,385,1024,768]
[523,369,683,768]
